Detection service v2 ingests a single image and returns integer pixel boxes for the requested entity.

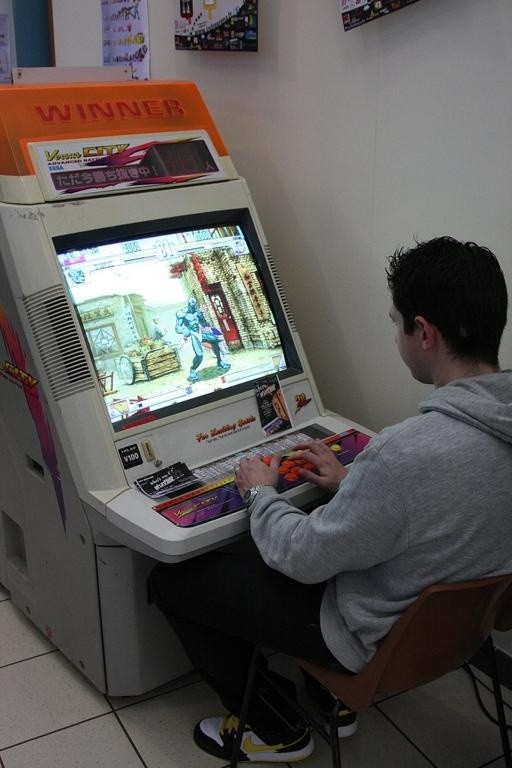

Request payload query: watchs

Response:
[242,483,273,507]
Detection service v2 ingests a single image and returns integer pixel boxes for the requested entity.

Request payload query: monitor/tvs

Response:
[58,224,288,423]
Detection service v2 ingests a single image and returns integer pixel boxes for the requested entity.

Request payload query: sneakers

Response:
[193,714,314,762]
[301,690,359,739]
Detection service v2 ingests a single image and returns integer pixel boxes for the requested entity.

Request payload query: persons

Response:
[175,298,231,381]
[148,236,511,762]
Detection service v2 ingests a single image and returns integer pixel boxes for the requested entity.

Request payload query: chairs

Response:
[155,574,512,768]
